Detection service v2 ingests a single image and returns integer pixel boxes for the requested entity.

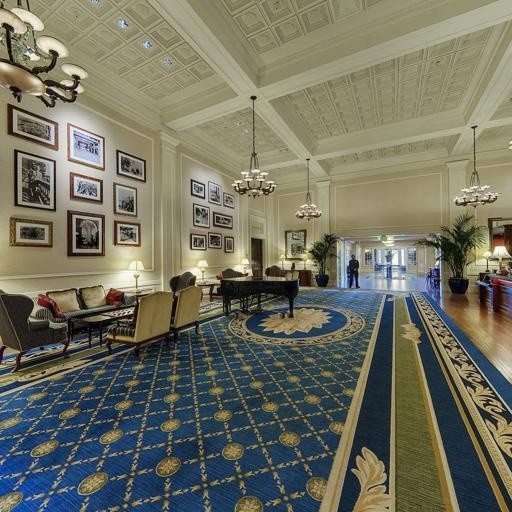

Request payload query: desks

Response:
[280,270,310,287]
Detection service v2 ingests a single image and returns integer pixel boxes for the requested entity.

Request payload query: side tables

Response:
[197,283,220,302]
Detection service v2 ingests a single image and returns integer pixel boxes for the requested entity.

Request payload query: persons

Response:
[348,254,361,289]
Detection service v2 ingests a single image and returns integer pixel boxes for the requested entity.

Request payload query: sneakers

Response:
[349,285,360,289]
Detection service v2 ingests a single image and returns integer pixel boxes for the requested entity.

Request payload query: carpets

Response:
[0,290,512,512]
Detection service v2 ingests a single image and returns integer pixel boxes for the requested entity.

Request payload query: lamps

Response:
[295,159,321,222]
[231,96,278,199]
[241,259,249,273]
[196,260,208,281]
[0,0,89,108]
[454,125,503,208]
[483,251,491,272]
[279,255,286,269]
[128,260,145,292]
[490,246,512,274]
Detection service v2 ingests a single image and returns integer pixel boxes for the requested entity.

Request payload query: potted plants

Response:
[413,212,488,294]
[308,231,345,287]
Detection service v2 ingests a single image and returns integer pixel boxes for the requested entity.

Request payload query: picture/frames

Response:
[6,103,146,256]
[191,179,235,253]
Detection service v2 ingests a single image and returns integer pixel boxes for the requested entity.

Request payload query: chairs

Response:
[221,265,281,279]
[0,272,203,374]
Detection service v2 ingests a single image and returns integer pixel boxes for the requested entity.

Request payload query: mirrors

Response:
[489,218,512,261]
[285,229,307,260]
